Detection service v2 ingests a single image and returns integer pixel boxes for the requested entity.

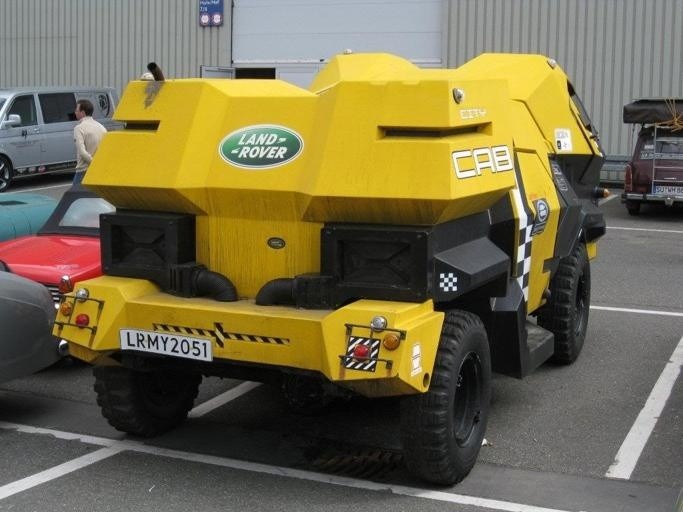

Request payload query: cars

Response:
[0,184,115,366]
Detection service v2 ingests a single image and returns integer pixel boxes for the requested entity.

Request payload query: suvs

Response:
[621,100,682,214]
[50,48,610,485]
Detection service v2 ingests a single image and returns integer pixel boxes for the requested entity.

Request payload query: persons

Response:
[70,99,107,188]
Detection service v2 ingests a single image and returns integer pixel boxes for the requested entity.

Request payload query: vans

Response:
[0,87,125,192]
